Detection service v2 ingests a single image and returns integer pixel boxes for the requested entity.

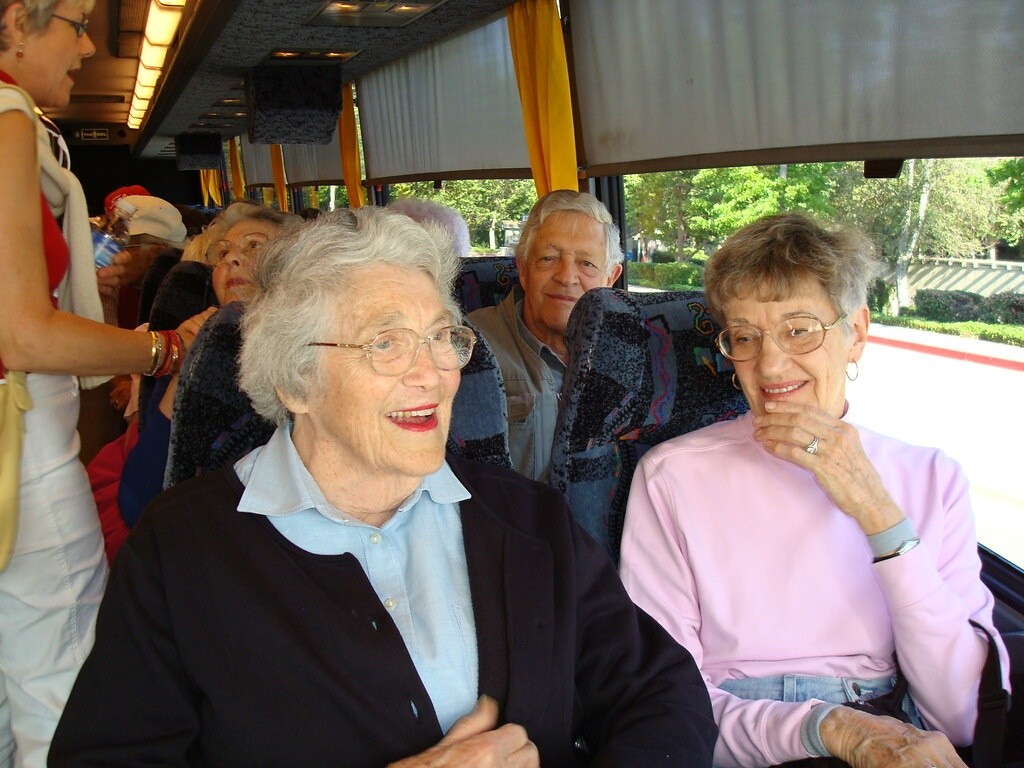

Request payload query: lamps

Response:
[126,0,187,130]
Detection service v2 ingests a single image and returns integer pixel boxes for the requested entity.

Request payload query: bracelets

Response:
[143,331,186,377]
[866,517,916,556]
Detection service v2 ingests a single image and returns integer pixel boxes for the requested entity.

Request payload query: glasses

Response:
[50,14,90,38]
[204,239,270,265]
[301,325,478,377]
[715,311,847,361]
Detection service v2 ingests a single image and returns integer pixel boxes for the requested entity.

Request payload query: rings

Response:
[805,437,819,454]
[113,400,118,408]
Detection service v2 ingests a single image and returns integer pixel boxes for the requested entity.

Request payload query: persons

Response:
[49,184,1011,768]
[0,0,215,768]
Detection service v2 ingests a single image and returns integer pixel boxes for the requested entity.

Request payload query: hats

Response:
[117,196,187,242]
[104,184,152,216]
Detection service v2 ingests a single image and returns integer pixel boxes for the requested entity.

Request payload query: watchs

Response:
[873,538,920,563]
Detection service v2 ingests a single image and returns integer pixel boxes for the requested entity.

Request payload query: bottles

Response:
[53,198,138,298]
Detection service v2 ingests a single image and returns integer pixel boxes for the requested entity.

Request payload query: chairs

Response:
[134,201,754,570]
